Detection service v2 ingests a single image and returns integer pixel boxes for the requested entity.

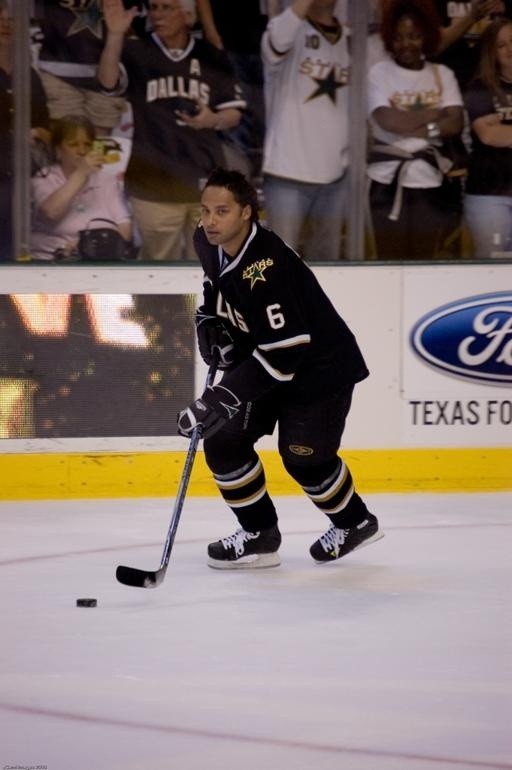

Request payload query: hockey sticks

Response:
[117,347,222,587]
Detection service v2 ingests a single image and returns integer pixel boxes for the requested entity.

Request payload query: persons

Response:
[1,0,512,261]
[175,170,378,563]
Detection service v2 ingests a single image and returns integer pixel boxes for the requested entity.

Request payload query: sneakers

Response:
[310,512,378,560]
[209,523,281,561]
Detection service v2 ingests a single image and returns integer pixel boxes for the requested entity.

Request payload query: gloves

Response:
[193,306,238,369]
[176,379,246,439]
